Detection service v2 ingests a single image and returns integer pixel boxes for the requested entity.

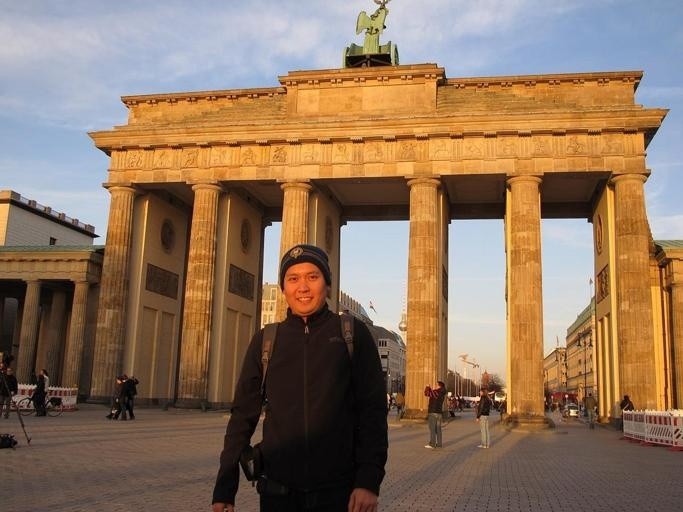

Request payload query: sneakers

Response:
[1,413,47,418]
[104,415,136,421]
[424,442,490,450]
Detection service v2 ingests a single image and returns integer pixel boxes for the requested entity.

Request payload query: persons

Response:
[387,392,507,422]
[32,369,49,417]
[476,389,491,449]
[620,395,634,432]
[586,393,595,429]
[424,381,447,448]
[212,245,388,512]
[105,374,139,421]
[0,367,18,419]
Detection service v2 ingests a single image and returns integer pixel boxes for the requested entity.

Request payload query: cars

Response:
[562,402,580,419]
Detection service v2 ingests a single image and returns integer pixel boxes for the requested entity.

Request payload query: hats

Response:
[280,244,331,291]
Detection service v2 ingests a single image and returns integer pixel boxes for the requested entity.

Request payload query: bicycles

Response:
[17,390,64,417]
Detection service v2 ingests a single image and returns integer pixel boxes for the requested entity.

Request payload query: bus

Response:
[494,391,506,409]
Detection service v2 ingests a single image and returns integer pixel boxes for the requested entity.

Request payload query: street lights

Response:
[577,332,594,397]
[555,349,567,393]
